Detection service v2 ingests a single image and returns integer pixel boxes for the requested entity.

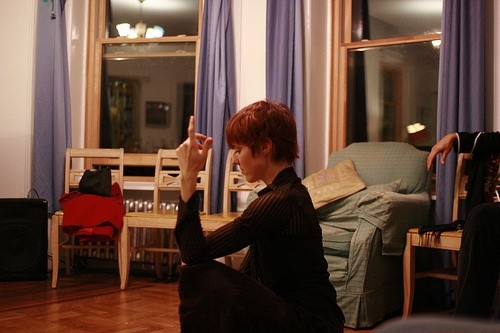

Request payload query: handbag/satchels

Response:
[77,167,112,197]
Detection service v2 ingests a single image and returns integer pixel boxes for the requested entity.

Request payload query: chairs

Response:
[120,149,213,287]
[402,153,473,320]
[196,149,260,255]
[51,148,124,288]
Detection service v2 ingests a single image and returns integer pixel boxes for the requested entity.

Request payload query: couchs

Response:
[316,141,433,329]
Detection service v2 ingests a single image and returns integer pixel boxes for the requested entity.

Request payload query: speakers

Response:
[0,198,48,282]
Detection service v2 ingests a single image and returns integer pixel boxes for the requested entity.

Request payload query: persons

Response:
[427,131,500,320]
[175,99,346,333]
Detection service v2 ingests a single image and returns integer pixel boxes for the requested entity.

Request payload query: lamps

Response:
[116,0,165,38]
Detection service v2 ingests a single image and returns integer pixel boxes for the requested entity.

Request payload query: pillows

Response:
[317,180,401,230]
[301,159,367,209]
